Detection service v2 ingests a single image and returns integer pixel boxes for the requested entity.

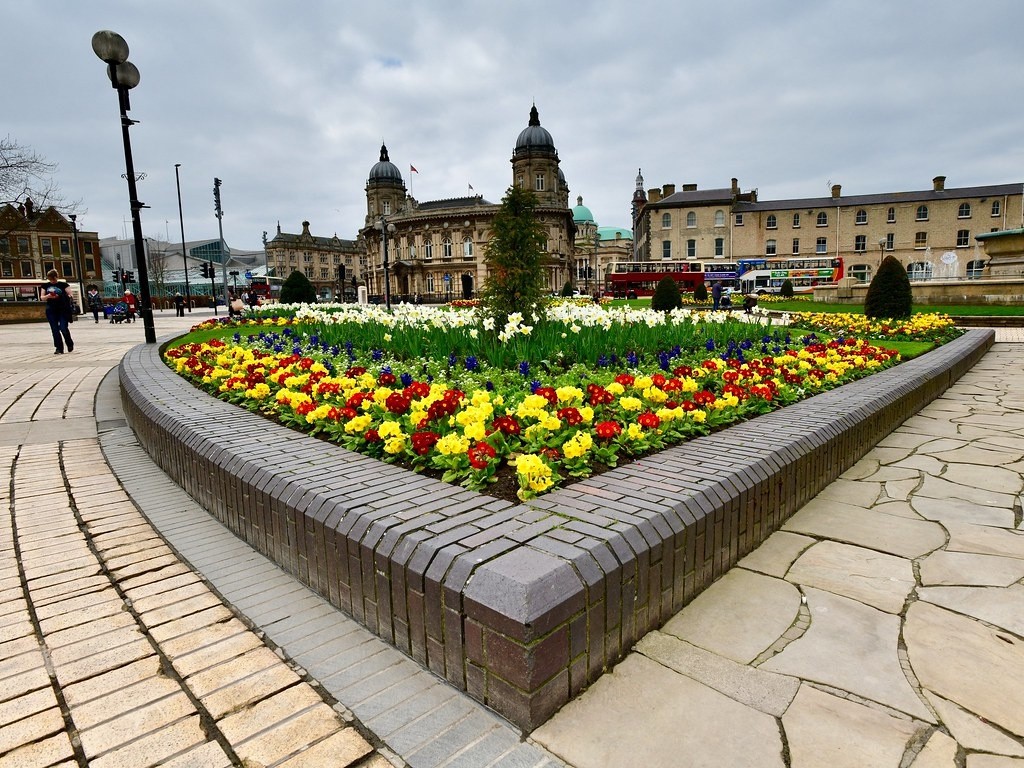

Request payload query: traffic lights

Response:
[126,270,136,283]
[588,267,593,278]
[200,262,208,278]
[574,268,577,276]
[112,271,120,283]
[579,268,583,278]
[121,269,126,283]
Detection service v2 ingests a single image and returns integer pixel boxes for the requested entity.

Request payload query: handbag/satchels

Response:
[130,304,135,309]
[179,302,183,306]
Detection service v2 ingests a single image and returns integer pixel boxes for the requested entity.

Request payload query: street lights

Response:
[593,232,602,292]
[374,214,397,312]
[879,239,886,262]
[68,215,87,314]
[90,28,158,345]
[175,164,192,312]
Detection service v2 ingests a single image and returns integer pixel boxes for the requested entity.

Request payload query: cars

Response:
[334,292,358,303]
[367,293,423,305]
[548,291,558,297]
[572,289,600,299]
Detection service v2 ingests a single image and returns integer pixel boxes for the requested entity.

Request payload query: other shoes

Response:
[125,320,131,323]
[54,347,63,355]
[68,341,73,352]
[133,315,135,321]
[95,320,99,323]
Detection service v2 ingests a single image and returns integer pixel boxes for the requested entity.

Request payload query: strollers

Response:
[110,301,132,324]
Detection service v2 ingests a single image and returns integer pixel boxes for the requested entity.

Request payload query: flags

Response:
[469,184,474,190]
[411,164,418,173]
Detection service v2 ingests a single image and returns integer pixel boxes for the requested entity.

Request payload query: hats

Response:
[124,289,130,294]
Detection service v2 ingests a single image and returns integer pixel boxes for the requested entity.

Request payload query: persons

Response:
[592,291,599,303]
[242,290,258,309]
[121,289,136,323]
[743,290,757,314]
[580,287,585,295]
[87,289,102,324]
[228,293,242,316]
[748,263,810,271]
[711,280,728,312]
[174,292,186,317]
[105,302,114,324]
[794,280,819,287]
[40,269,74,354]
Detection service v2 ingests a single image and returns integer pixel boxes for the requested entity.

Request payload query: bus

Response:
[737,256,845,296]
[0,278,67,302]
[704,262,740,291]
[251,274,288,301]
[603,261,705,298]
